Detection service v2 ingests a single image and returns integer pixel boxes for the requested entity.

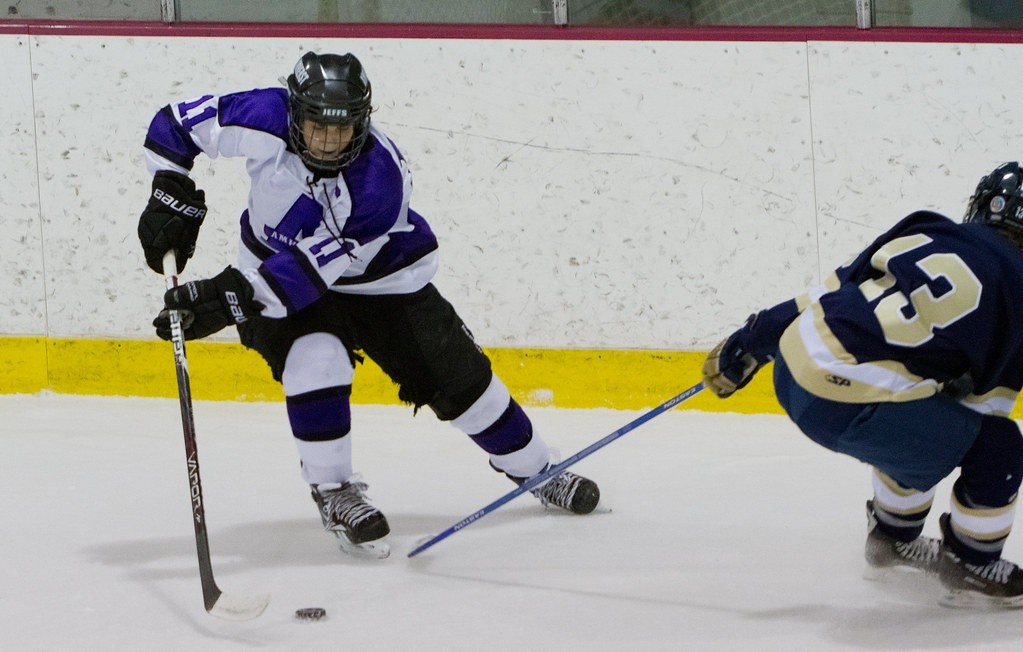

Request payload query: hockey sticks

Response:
[161,248,270,621]
[405,377,708,558]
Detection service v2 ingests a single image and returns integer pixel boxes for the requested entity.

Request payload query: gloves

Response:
[153,264,255,342]
[701,308,777,399]
[138,170,208,274]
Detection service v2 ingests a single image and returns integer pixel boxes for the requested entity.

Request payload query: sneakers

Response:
[489,458,612,516]
[299,460,391,559]
[939,511,1023,608]
[862,499,941,581]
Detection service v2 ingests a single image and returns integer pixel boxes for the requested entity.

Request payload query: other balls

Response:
[294,608,327,621]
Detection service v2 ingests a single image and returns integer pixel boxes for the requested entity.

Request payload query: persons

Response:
[136,51,600,541]
[702,159,1023,608]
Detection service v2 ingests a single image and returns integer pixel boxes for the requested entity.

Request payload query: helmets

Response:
[286,51,373,171]
[962,162,1023,255]
